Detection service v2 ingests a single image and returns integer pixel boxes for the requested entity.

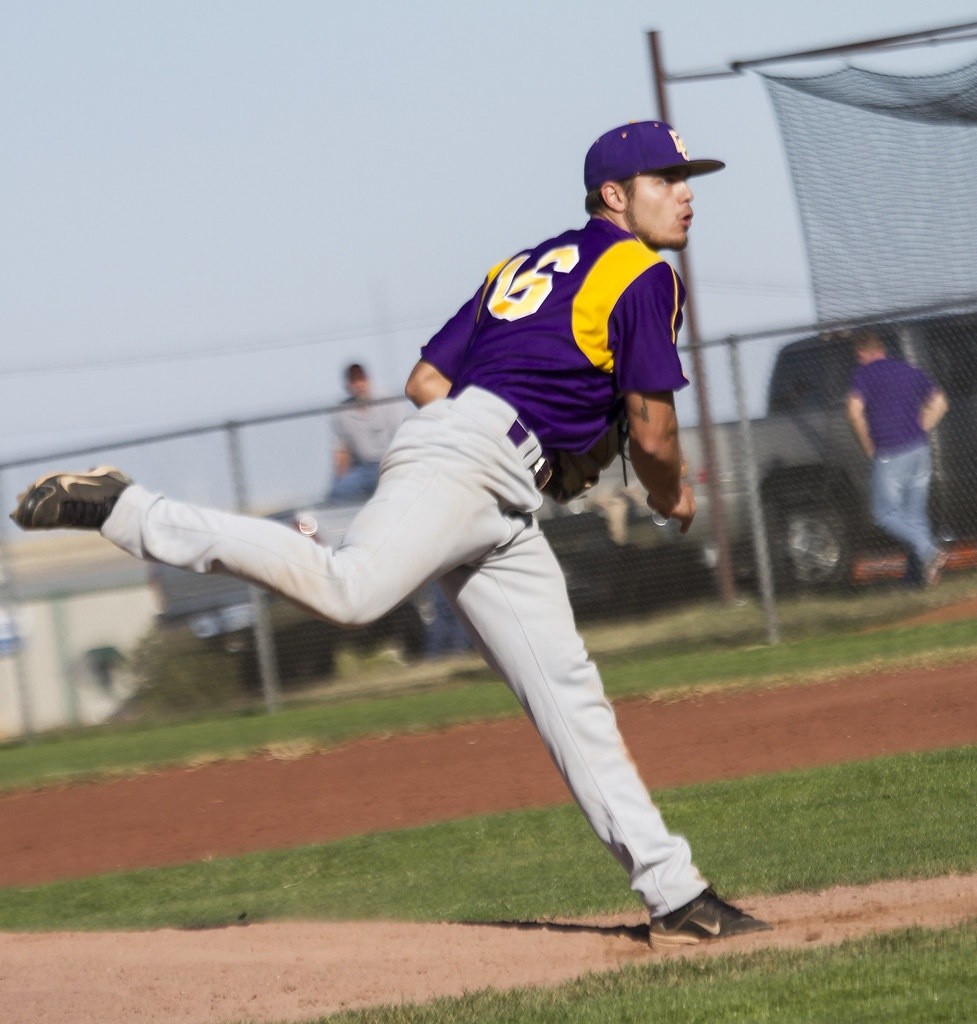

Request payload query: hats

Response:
[345,363,366,382]
[583,121,726,193]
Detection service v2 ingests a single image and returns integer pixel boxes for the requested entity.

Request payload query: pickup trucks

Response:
[539,309,975,611]
[148,491,468,689]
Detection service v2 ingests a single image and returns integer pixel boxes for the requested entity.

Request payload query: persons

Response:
[841,326,954,593]
[9,120,774,951]
[325,361,397,501]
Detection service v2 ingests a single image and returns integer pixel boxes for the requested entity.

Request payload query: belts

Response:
[449,388,552,491]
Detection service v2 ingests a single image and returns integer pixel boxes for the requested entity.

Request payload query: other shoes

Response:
[919,549,952,590]
[886,579,918,594]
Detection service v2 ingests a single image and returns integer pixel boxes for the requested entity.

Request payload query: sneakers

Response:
[650,883,773,951]
[10,466,130,532]
